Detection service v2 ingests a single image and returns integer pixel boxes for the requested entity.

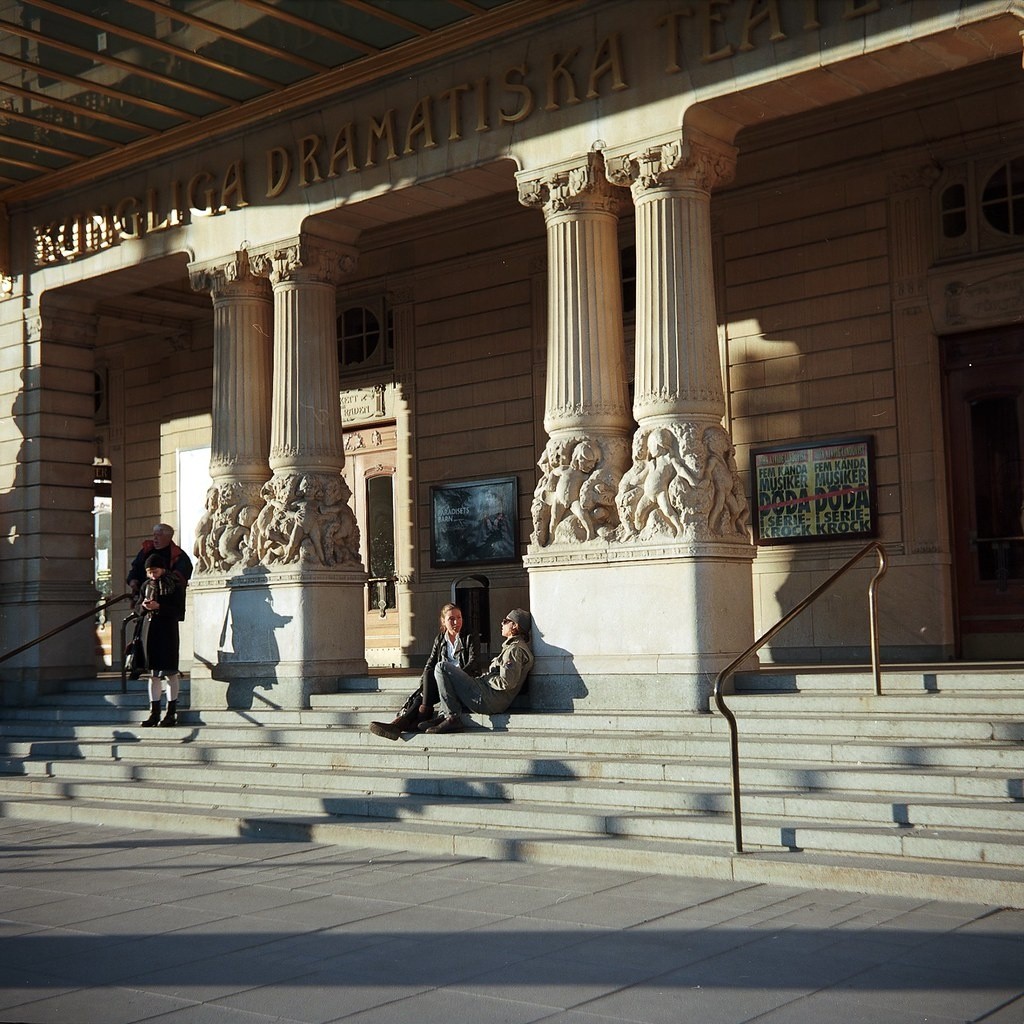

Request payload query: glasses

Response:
[503,618,514,625]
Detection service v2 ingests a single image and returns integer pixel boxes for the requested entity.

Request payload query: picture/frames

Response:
[749,434,881,547]
[429,475,522,569]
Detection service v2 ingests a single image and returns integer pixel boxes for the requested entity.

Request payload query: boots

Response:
[141,700,161,727]
[368,717,406,740]
[158,699,181,726]
[403,706,434,733]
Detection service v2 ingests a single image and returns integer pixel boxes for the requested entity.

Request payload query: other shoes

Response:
[418,714,447,732]
[426,716,464,734]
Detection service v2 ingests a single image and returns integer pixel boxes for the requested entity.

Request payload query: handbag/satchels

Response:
[125,636,140,672]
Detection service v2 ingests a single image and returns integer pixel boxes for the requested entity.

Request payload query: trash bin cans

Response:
[451,574,491,663]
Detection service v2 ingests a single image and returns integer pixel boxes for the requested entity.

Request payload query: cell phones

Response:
[145,602,150,605]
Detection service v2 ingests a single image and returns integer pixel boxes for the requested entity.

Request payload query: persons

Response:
[369,603,481,741]
[126,523,193,681]
[531,426,751,546]
[418,607,533,734]
[135,554,186,728]
[193,475,361,573]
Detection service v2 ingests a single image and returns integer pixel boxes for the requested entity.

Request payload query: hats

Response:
[507,608,532,632]
[144,555,166,569]
